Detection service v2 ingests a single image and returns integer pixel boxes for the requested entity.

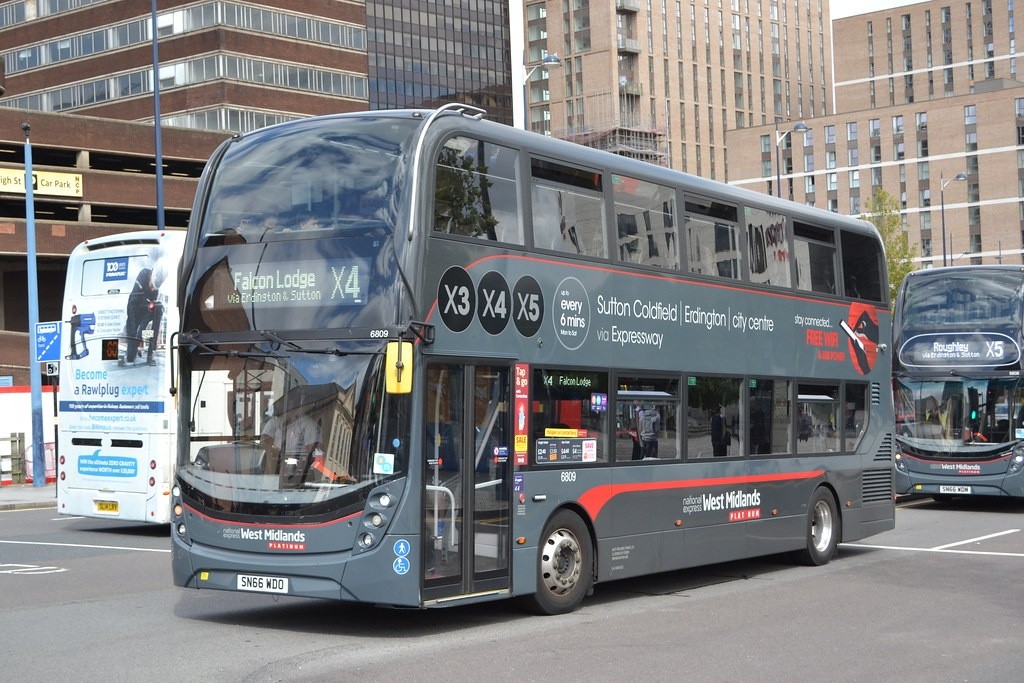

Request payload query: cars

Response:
[988,404,1020,426]
[895,405,918,438]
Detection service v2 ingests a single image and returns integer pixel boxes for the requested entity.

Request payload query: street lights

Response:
[775,124,808,199]
[21,121,46,485]
[941,173,967,266]
[523,56,563,130]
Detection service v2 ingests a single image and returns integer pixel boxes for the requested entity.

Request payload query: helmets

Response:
[152,265,168,289]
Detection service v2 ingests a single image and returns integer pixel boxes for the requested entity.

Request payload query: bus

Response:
[894,265,1024,505]
[168,101,894,613]
[53,230,272,525]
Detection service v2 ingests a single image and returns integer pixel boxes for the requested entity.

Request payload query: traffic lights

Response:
[968,388,979,425]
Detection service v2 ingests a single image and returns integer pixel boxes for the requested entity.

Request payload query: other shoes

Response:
[147,357,156,366]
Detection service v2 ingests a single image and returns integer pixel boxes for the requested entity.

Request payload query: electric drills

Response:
[65,312,96,358]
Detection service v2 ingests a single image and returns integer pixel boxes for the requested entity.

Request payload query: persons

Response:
[259,210,318,232]
[750,396,764,454]
[260,389,320,474]
[710,403,731,456]
[126,264,168,367]
[631,403,660,460]
